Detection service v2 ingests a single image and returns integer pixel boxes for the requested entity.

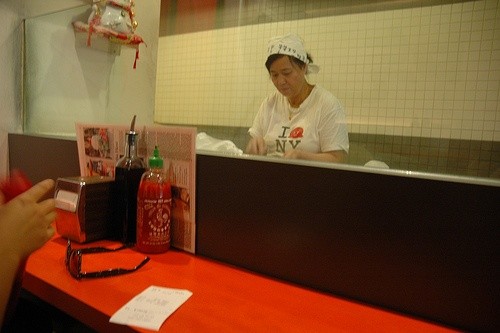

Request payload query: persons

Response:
[245,31,349,164]
[0,179,57,332]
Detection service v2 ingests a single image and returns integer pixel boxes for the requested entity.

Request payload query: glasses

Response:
[65,240,149,279]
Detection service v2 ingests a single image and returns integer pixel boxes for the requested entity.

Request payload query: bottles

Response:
[135,144,172,254]
[113,114,150,244]
